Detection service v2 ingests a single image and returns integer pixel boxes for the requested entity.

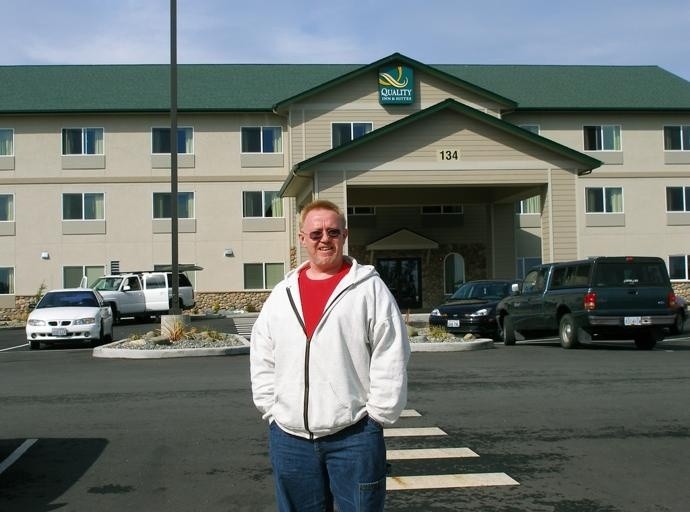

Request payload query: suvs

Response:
[495,255,679,351]
[79,272,197,326]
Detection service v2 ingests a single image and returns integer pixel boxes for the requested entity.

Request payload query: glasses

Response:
[301,228,343,241]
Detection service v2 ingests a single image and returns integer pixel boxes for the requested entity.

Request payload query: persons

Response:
[250,201,408,511]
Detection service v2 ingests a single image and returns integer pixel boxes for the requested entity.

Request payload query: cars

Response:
[25,288,114,349]
[662,295,690,335]
[429,279,522,341]
